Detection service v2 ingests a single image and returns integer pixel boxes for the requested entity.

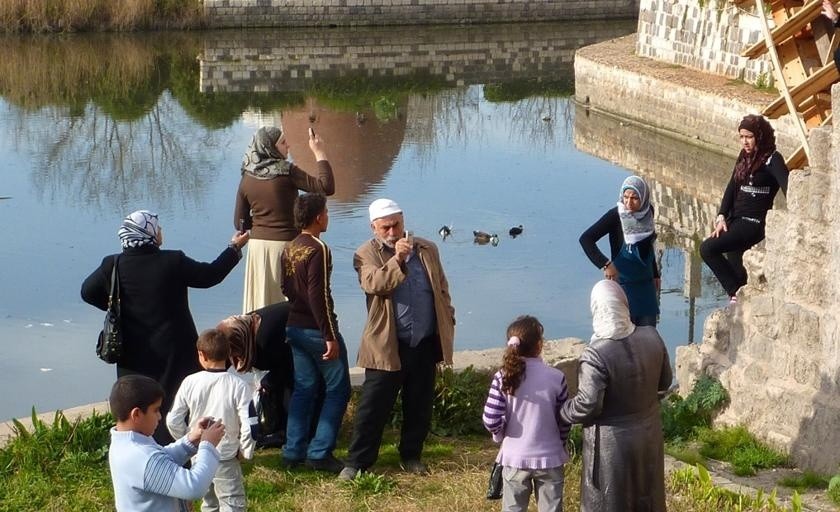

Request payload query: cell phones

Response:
[239,217,246,235]
[405,229,414,248]
[308,127,315,139]
[206,418,216,430]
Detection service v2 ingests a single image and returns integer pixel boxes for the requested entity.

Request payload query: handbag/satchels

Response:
[95,252,127,363]
[487,461,503,500]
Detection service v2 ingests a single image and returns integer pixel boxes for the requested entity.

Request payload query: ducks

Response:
[508,222,525,241]
[472,228,500,248]
[436,220,457,242]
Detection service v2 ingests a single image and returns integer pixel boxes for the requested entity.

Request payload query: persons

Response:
[483,314,571,512]
[820,0,840,28]
[80,209,250,470]
[700,114,789,304]
[255,369,295,446]
[234,126,335,314]
[559,279,673,512]
[216,301,292,371]
[579,175,660,328]
[109,374,225,512]
[166,328,256,512]
[280,191,352,472]
[337,198,456,482]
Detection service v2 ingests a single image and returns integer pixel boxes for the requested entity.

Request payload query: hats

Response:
[370,199,403,222]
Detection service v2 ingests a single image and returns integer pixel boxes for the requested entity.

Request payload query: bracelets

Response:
[604,261,612,271]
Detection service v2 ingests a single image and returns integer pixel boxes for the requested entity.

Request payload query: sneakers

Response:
[400,455,424,474]
[307,453,342,474]
[339,467,360,478]
